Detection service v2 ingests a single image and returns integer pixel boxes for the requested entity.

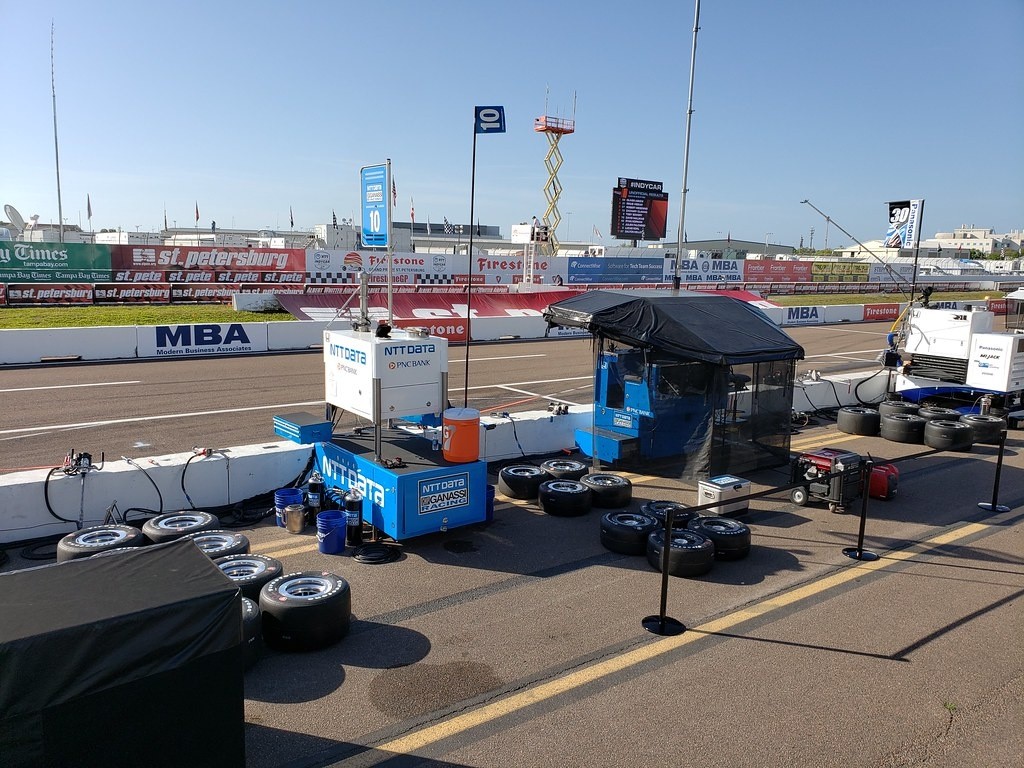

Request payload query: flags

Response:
[427,217,431,236]
[196,204,199,222]
[88,196,92,220]
[333,211,337,226]
[938,243,942,253]
[444,216,453,235]
[728,231,730,243]
[685,231,688,244]
[477,222,481,237]
[291,211,294,227]
[392,179,397,207]
[411,204,415,224]
[958,245,961,251]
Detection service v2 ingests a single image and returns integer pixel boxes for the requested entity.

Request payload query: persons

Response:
[594,226,602,239]
[532,216,539,240]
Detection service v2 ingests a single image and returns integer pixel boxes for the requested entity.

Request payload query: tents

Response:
[543,289,804,480]
[1002,290,1024,329]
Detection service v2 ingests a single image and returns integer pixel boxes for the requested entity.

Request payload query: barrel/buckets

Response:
[442,407,480,463]
[316,510,348,554]
[274,488,303,527]
[281,504,309,534]
